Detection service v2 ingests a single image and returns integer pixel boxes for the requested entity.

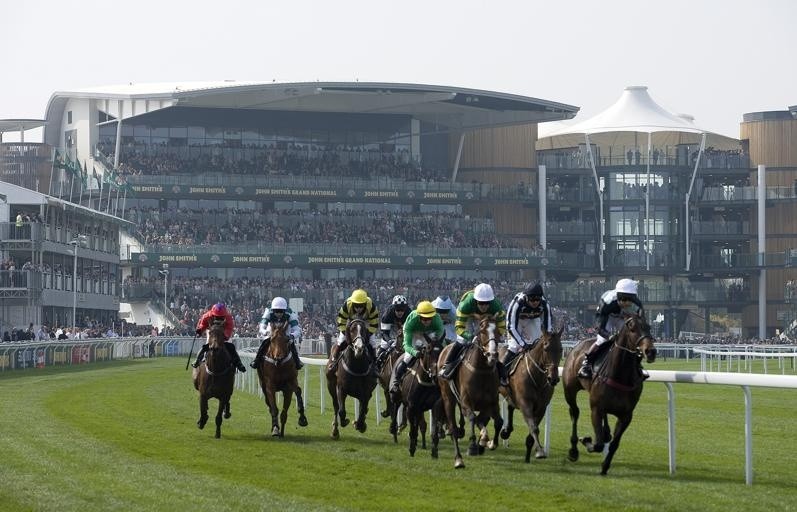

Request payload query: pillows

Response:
[271,297,287,311]
[473,284,495,302]
[392,296,406,304]
[433,296,451,311]
[523,282,543,297]
[417,301,436,318]
[615,279,639,294]
[212,304,228,316]
[351,290,368,304]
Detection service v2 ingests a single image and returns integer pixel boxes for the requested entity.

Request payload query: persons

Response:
[630,214,637,235]
[635,150,642,165]
[2,323,197,367]
[14,211,117,246]
[213,276,273,338]
[653,333,797,359]
[516,176,754,202]
[251,296,303,370]
[692,147,746,169]
[479,276,553,311]
[390,301,443,392]
[0,258,116,290]
[191,304,246,372]
[375,294,413,373]
[537,148,594,168]
[432,296,458,345]
[577,279,649,380]
[537,214,600,236]
[626,148,633,166]
[352,277,416,317]
[417,278,479,307]
[658,148,665,165]
[322,330,332,358]
[124,274,167,298]
[652,148,659,165]
[501,281,552,385]
[328,289,379,373]
[443,285,506,379]
[273,276,351,339]
[552,306,599,341]
[727,282,752,303]
[168,276,213,335]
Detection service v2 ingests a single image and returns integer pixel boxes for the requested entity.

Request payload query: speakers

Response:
[250,347,265,368]
[497,361,509,387]
[328,345,340,372]
[231,351,246,372]
[292,350,302,369]
[376,347,386,372]
[639,363,649,381]
[503,349,516,366]
[443,341,463,379]
[372,349,379,378]
[191,349,205,368]
[389,361,408,393]
[578,343,601,378]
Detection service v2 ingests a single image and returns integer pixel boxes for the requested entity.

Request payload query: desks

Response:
[158,269,169,337]
[69,238,79,339]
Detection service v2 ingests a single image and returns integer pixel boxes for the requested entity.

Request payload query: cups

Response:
[528,297,541,302]
[274,310,284,313]
[354,306,364,308]
[476,302,490,305]
[213,318,225,321]
[395,309,406,312]
[420,318,433,321]
[619,296,634,302]
[438,310,450,313]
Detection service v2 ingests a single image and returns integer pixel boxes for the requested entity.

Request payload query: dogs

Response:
[379,325,405,435]
[562,308,656,476]
[389,329,447,460]
[193,318,235,439]
[327,311,377,441]
[499,323,564,463]
[257,320,308,441]
[437,312,504,469]
